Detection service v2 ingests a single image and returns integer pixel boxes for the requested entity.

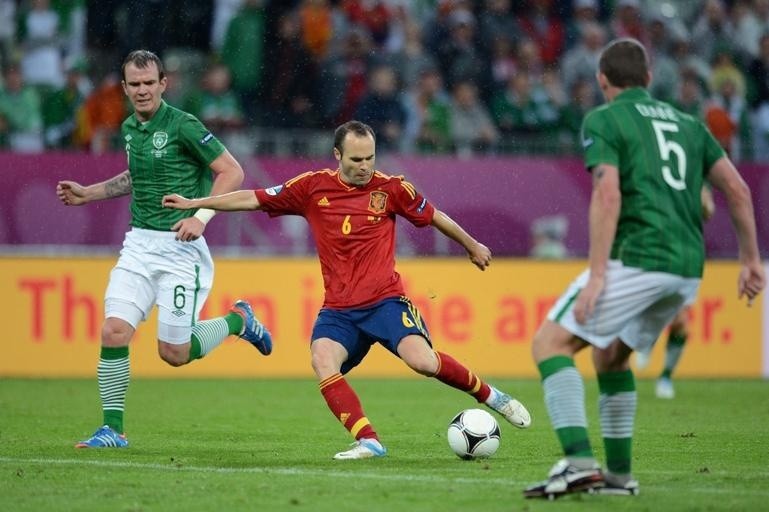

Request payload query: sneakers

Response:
[77,425,127,449]
[523,457,639,499]
[483,386,531,428]
[333,438,386,461]
[229,299,271,356]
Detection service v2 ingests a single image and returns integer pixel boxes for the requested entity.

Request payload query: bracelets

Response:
[193,206,216,226]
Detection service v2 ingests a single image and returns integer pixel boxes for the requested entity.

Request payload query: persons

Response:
[160,119,530,461]
[55,48,273,449]
[634,179,716,399]
[517,39,765,499]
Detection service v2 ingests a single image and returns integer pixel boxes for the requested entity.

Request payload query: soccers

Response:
[447,408,501,461]
